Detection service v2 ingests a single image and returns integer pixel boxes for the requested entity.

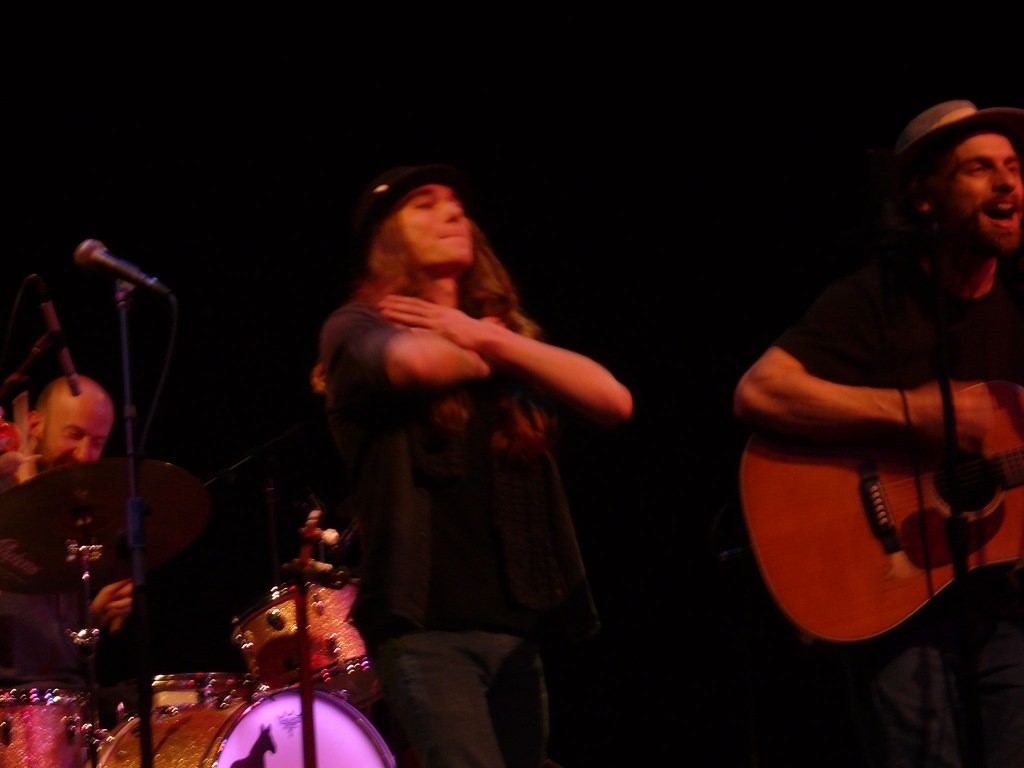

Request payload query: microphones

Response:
[74,239,172,296]
[34,274,82,396]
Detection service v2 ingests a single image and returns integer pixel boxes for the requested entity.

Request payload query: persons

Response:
[317,162,633,768]
[732,98,1024,768]
[0,374,136,695]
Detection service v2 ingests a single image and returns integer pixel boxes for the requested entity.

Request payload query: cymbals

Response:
[0,458,209,595]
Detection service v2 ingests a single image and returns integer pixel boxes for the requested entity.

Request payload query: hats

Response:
[881,99,1024,199]
[345,163,478,274]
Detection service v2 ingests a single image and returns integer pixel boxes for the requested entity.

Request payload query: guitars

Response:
[737,375,1024,643]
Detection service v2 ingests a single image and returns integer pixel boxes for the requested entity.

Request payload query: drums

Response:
[86,686,397,768]
[231,578,372,695]
[152,673,244,708]
[0,688,99,768]
